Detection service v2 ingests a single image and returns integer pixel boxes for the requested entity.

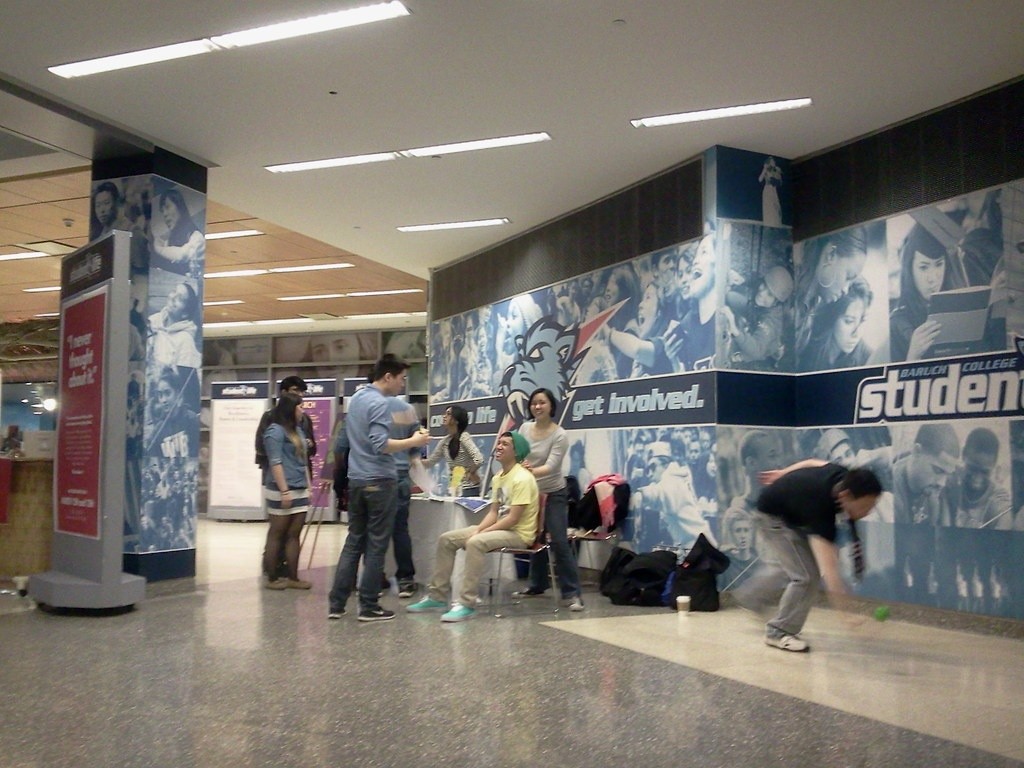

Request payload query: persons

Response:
[511,387,585,611]
[731,457,882,654]
[141,458,196,552]
[153,189,206,278]
[147,377,200,456]
[89,180,149,270]
[306,331,377,379]
[724,156,792,370]
[252,375,317,590]
[614,427,761,593]
[430,217,718,402]
[405,431,541,621]
[714,424,1015,530]
[201,339,237,444]
[419,405,485,496]
[567,440,593,501]
[145,281,202,369]
[795,196,1009,369]
[327,354,433,620]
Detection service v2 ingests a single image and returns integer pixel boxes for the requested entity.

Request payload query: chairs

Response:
[565,473,631,591]
[484,492,559,618]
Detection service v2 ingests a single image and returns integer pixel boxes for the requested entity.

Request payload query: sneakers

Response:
[442,600,476,622]
[398,576,414,598]
[285,576,311,589]
[357,606,396,621]
[568,596,584,611]
[258,574,286,590]
[511,587,543,598]
[329,607,347,618]
[376,575,392,596]
[405,596,448,613]
[762,630,808,652]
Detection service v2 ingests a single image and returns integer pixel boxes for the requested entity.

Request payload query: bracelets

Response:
[281,490,289,496]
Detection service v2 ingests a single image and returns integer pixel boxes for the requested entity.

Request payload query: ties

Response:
[847,517,863,580]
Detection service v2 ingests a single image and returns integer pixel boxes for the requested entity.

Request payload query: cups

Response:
[420,427,429,434]
[676,596,690,615]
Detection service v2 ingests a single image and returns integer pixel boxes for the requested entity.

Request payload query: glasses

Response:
[644,462,666,475]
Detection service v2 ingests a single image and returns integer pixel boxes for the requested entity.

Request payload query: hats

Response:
[507,430,531,462]
[643,441,672,458]
[816,428,849,460]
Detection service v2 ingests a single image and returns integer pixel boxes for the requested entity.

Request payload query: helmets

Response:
[765,265,793,303]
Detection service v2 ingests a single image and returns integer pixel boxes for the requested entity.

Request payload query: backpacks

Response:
[575,479,632,536]
[598,546,677,605]
[564,475,581,527]
[671,533,729,611]
